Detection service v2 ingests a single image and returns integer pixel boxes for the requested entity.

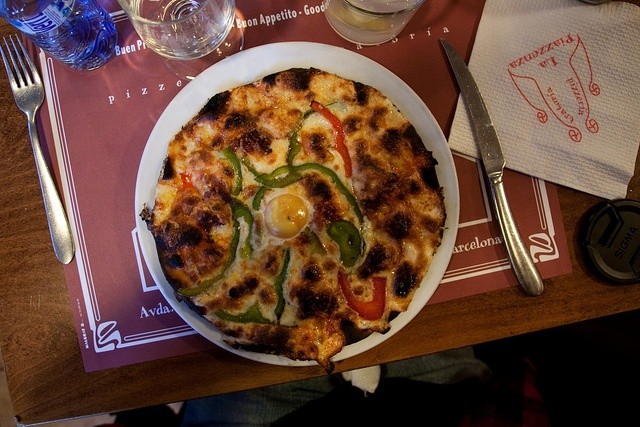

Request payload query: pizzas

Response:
[139,67,447,375]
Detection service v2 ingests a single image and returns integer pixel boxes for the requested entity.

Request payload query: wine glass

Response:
[117,0,245,83]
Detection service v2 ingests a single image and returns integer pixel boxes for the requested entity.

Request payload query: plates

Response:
[133,40,461,369]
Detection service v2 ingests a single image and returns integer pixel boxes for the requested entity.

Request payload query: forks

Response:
[0,33,76,264]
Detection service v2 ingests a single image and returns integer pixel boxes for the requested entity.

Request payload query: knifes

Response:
[439,36,545,297]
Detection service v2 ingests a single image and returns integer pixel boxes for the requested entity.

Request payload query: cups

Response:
[322,0,427,46]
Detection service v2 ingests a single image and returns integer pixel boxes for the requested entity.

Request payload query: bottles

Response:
[1,0,121,72]
